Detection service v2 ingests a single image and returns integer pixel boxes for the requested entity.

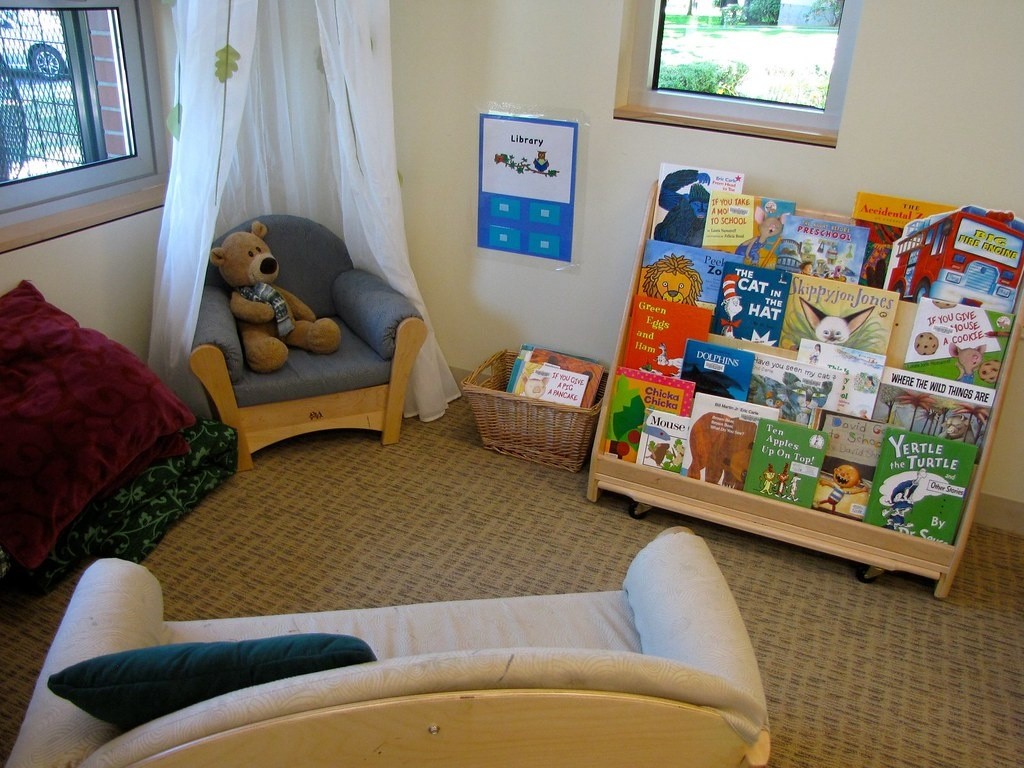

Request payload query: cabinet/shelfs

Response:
[586,179,1024,601]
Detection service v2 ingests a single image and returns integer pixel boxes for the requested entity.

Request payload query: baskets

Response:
[459,348,608,473]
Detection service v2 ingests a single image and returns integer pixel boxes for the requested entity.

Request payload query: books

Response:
[506,343,605,409]
[602,160,1023,549]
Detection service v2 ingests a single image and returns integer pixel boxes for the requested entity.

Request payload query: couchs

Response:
[182,214,430,473]
[5,525,773,767]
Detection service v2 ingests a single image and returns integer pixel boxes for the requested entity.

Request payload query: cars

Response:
[0,9,70,81]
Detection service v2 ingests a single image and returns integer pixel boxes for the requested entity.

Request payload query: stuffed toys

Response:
[208,221,341,373]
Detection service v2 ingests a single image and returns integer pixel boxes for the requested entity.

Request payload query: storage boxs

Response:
[460,347,609,473]
